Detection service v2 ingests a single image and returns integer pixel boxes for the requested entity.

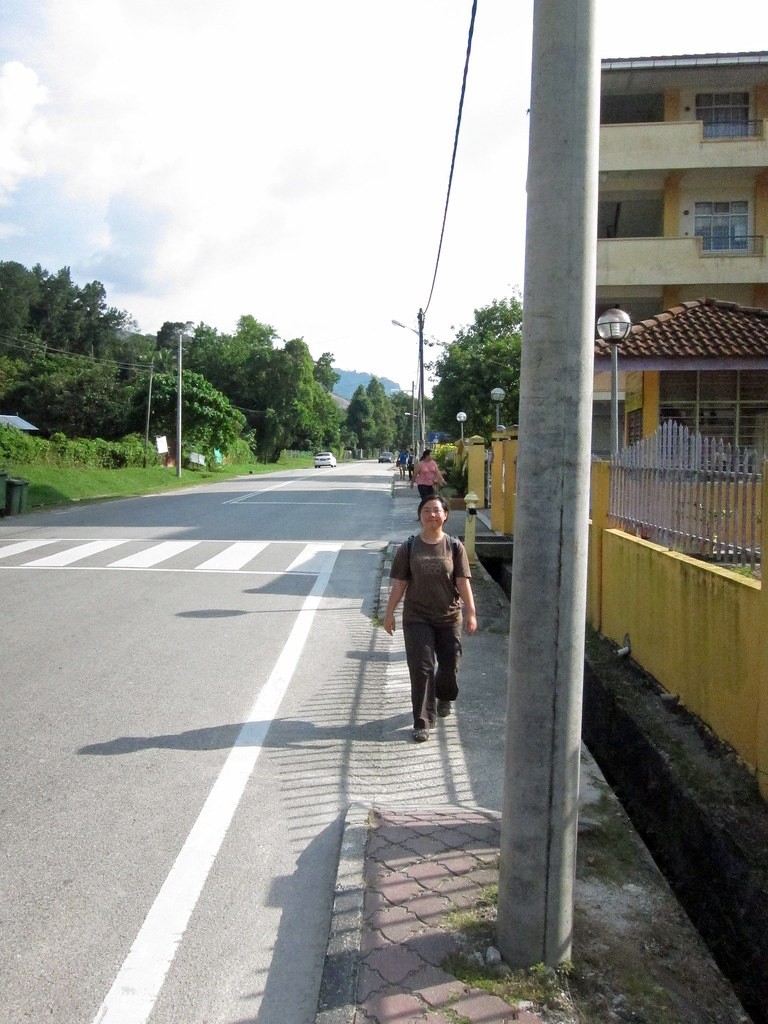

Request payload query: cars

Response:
[313,452,336,468]
[378,451,395,463]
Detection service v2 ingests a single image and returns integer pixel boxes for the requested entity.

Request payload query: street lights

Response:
[490,387,506,436]
[391,389,415,449]
[456,411,467,443]
[596,308,632,459]
[177,321,195,478]
[404,413,420,429]
[392,319,425,407]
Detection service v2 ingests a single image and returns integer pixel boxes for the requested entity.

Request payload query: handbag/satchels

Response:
[396,461,400,467]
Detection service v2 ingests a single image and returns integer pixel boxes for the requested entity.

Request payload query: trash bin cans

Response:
[16,476,30,512]
[6,477,23,517]
[0,468,8,518]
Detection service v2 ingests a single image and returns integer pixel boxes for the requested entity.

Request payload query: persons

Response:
[395,448,415,480]
[409,449,447,501]
[381,495,479,744]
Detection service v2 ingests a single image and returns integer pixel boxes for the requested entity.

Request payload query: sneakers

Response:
[412,728,429,741]
[437,699,451,717]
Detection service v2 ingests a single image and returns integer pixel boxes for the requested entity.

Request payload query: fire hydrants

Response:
[463,490,479,566]
